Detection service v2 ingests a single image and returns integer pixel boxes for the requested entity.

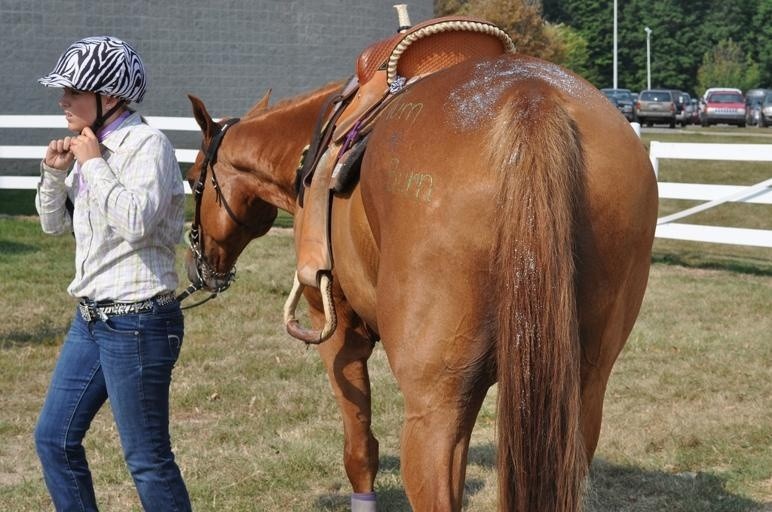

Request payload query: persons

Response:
[36,35,192,512]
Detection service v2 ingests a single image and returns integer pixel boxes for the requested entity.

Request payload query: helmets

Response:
[38,37,146,103]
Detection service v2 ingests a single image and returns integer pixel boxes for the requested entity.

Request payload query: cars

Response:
[600,87,772,128]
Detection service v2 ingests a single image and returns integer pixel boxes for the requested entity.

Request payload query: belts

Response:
[80,291,174,322]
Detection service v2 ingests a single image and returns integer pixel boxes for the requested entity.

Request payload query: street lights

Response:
[644,27,652,89]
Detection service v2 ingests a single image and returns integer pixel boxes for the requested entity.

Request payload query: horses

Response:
[184,55,659,512]
